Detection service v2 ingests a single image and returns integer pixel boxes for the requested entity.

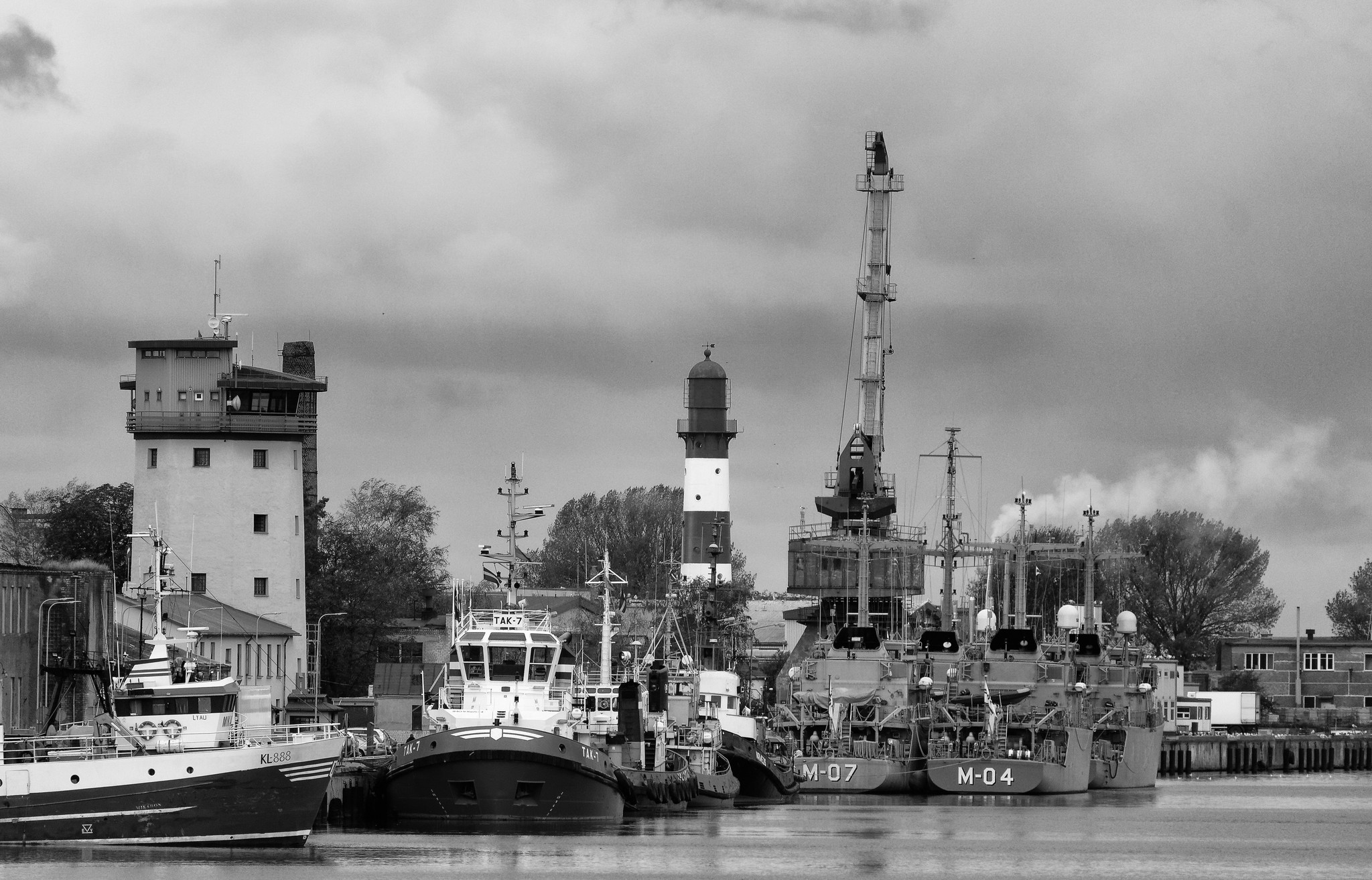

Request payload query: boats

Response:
[780,129,933,793]
[351,452,633,823]
[927,474,1101,794]
[0,499,350,849]
[918,427,1000,706]
[569,505,808,834]
[1039,489,1165,789]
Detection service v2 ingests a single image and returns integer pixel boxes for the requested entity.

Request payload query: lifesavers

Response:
[164,720,182,739]
[138,721,156,740]
